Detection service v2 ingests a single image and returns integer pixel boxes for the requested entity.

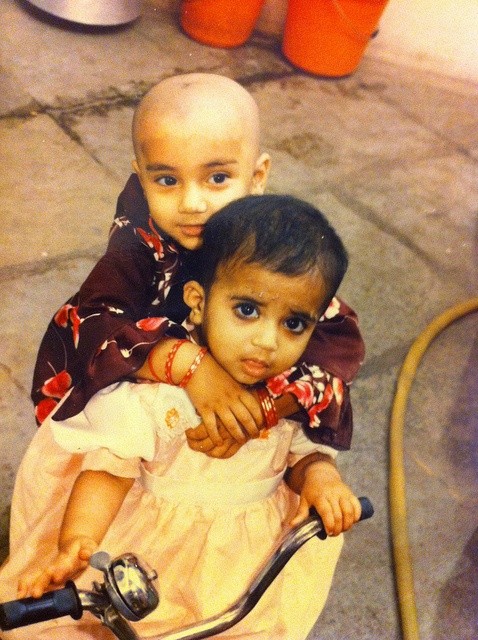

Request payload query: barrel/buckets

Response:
[282,0,389,78]
[179,0,265,48]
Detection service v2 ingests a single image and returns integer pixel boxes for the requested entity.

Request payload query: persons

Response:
[1,194,362,640]
[32,73,367,459]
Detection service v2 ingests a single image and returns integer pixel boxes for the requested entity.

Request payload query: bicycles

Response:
[1,497,375,640]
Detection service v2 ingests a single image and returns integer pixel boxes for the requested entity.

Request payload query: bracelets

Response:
[256,385,279,429]
[146,333,205,387]
